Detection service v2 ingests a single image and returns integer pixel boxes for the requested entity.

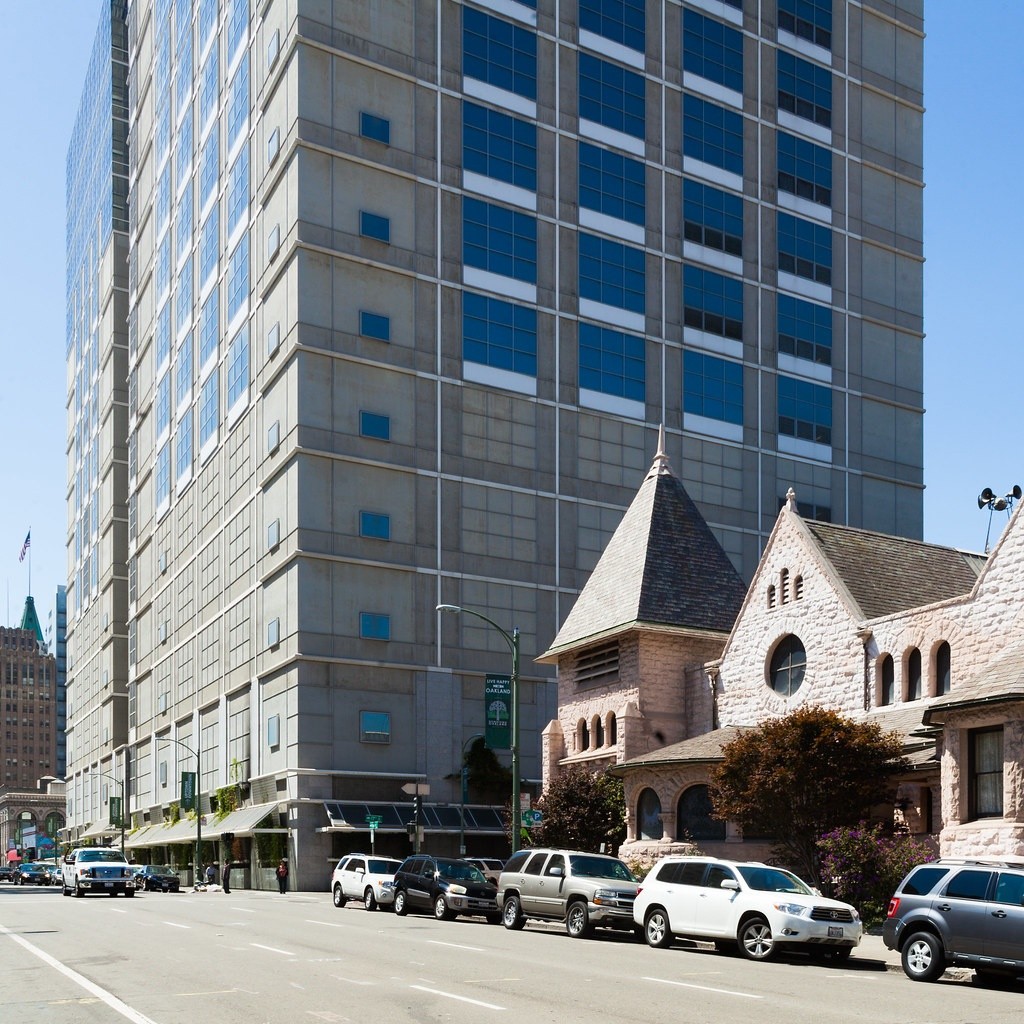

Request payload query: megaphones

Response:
[977,487,997,509]
[1006,485,1023,499]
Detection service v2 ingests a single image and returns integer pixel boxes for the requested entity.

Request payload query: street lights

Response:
[30,799,58,863]
[155,736,204,890]
[435,604,522,856]
[87,773,125,855]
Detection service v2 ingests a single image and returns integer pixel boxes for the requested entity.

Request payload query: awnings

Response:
[79,818,123,838]
[106,799,292,849]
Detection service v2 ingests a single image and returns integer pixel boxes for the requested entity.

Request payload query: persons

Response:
[385,862,396,873]
[205,863,215,885]
[708,868,768,890]
[24,867,27,871]
[158,868,164,874]
[276,860,288,894]
[223,858,231,894]
[458,868,471,879]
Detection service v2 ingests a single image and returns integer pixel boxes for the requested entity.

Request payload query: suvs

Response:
[61,847,136,898]
[394,854,502,926]
[330,853,404,911]
[882,856,1024,989]
[453,857,505,885]
[496,847,641,939]
[632,855,864,964]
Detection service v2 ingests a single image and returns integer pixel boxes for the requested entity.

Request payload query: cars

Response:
[134,865,180,893]
[0,863,63,886]
[129,864,144,891]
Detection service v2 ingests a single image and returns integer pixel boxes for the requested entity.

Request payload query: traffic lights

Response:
[414,795,423,815]
